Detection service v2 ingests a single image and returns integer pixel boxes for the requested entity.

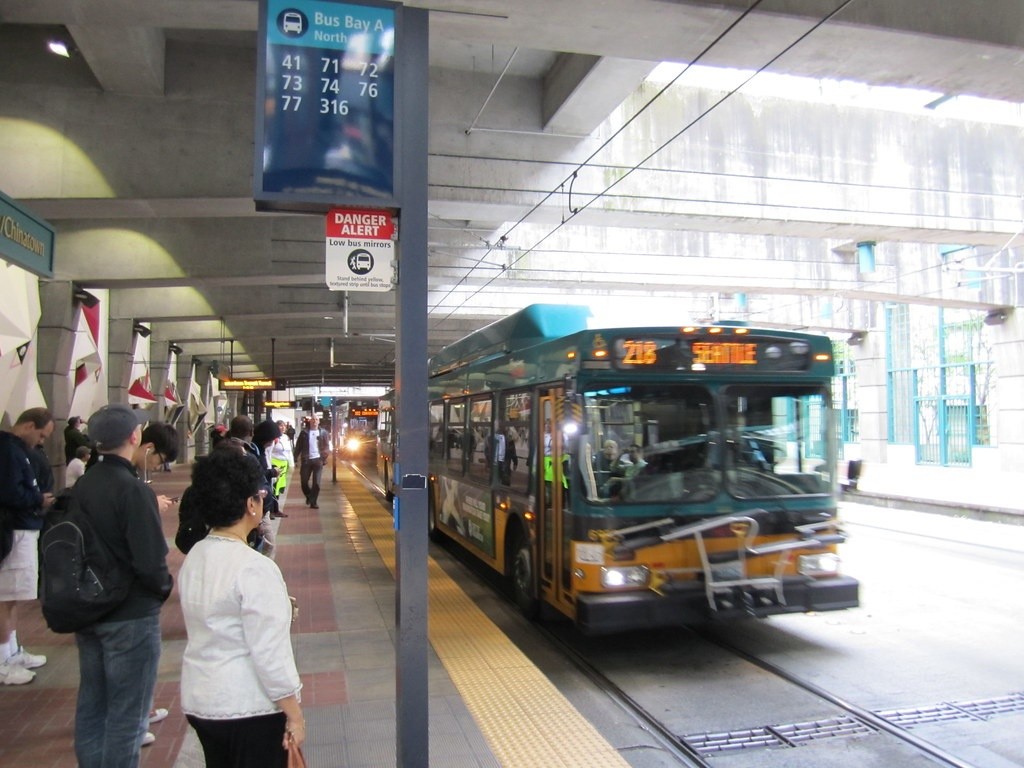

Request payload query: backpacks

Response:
[37,468,126,633]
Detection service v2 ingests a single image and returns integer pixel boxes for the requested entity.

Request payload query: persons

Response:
[129,423,180,745]
[293,416,332,509]
[0,407,57,685]
[208,414,296,558]
[428,414,568,506]
[590,440,650,501]
[30,415,98,492]
[54,404,173,768]
[176,447,306,768]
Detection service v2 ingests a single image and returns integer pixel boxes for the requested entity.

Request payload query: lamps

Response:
[985,312,1008,325]
[133,324,152,337]
[169,345,182,354]
[847,334,863,347]
[74,290,99,308]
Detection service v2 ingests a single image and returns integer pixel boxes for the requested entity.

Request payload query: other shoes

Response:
[306,498,310,505]
[310,505,318,509]
[275,512,288,517]
[149,708,168,723]
[270,513,275,520]
[142,732,155,746]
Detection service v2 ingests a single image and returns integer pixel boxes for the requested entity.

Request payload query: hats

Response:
[215,426,228,432]
[87,404,154,451]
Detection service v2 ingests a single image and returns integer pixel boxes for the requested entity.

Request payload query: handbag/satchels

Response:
[323,460,328,465]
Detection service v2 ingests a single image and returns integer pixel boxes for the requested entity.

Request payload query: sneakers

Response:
[0,645,47,685]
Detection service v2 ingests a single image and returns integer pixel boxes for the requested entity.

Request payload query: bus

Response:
[375,305,861,636]
[329,398,378,461]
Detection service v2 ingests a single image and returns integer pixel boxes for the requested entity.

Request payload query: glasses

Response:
[158,452,166,465]
